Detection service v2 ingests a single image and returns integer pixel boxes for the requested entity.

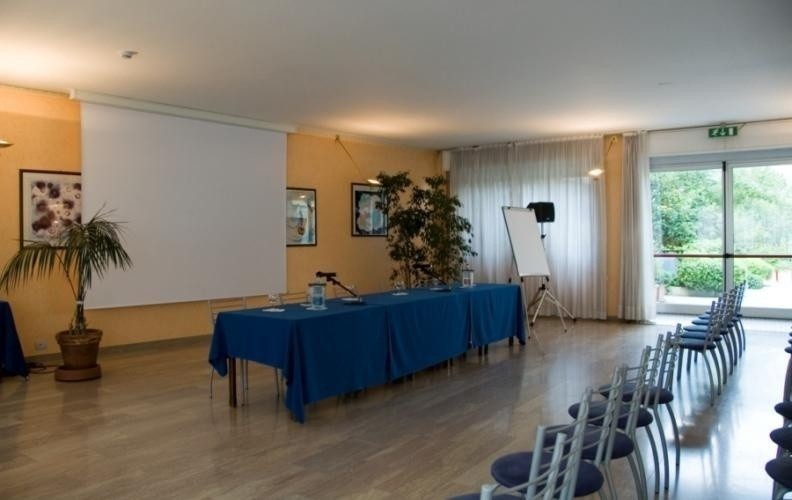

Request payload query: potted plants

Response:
[0,202,132,381]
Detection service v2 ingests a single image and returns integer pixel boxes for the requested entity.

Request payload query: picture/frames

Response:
[19,169,81,250]
[352,183,391,236]
[286,187,318,246]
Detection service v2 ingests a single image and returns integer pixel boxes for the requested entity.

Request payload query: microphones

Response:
[315,270,337,278]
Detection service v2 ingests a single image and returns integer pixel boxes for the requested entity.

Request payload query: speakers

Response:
[527,201,555,222]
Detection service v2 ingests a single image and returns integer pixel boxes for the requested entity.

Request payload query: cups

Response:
[463,268,474,287]
[267,291,283,310]
[311,285,325,309]
[393,275,407,294]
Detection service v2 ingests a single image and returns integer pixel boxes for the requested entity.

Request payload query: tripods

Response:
[527,231,576,327]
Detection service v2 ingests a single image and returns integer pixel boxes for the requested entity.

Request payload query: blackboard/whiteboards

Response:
[502,206,551,277]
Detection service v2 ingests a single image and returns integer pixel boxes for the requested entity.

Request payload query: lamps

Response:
[587,137,617,181]
[334,136,381,186]
